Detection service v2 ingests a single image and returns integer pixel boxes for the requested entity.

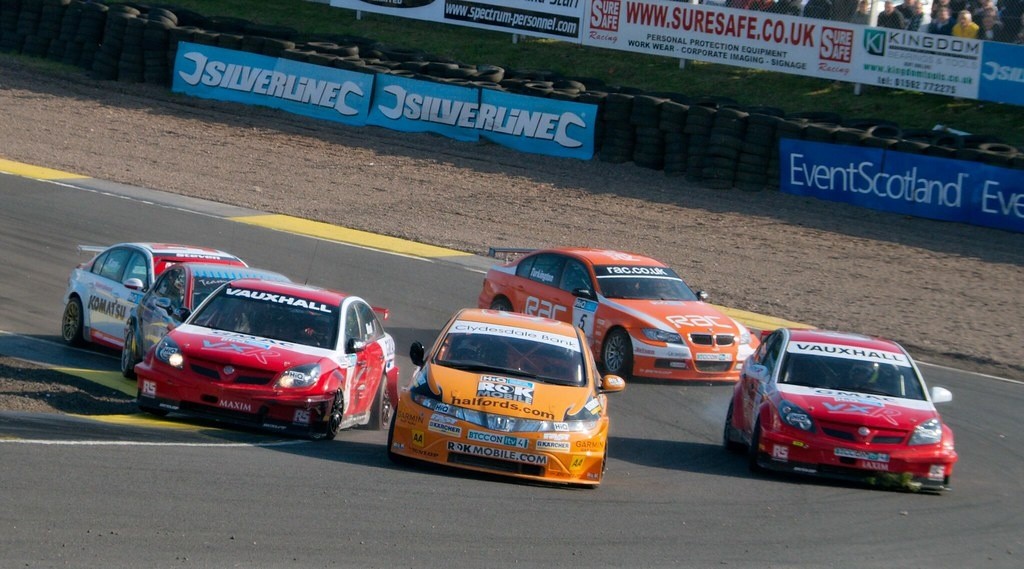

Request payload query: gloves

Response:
[305,328,314,336]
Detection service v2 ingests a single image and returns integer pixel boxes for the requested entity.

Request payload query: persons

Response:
[877,0,1024,45]
[302,312,329,342]
[845,361,879,390]
[702,0,870,25]
[455,338,486,362]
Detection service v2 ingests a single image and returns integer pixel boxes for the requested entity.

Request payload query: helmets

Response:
[850,363,879,383]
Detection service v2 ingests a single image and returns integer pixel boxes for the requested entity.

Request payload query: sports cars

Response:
[61,242,249,349]
[478,246,761,385]
[132,277,399,443]
[723,326,958,493]
[120,261,296,380]
[385,307,625,489]
